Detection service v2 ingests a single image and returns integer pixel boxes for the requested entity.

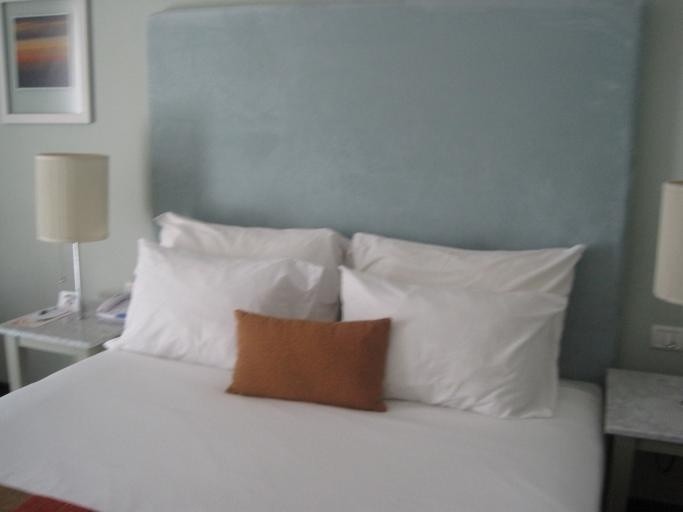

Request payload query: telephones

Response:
[94,292,130,322]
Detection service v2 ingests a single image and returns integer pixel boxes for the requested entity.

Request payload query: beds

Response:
[0,0,645,512]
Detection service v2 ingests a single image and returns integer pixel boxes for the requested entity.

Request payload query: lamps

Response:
[35,154,108,321]
[652,181,683,307]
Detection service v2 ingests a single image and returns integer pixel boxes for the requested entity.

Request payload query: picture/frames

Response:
[0,0,93,124]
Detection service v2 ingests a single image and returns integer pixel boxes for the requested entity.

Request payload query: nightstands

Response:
[603,368,683,512]
[0,306,123,392]
